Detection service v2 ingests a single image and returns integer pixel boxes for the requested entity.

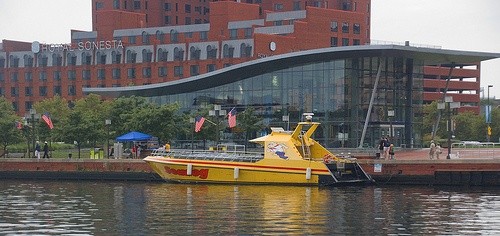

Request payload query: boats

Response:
[142,119,378,187]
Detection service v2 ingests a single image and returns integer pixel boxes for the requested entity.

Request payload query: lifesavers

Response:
[324,155,332,163]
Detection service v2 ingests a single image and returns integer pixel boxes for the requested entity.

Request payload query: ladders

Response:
[301,131,311,158]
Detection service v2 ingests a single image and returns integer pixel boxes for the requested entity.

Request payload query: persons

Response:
[165,143,170,158]
[378,136,395,159]
[35,141,41,159]
[132,144,141,159]
[428,140,435,159]
[43,141,49,158]
[435,143,441,160]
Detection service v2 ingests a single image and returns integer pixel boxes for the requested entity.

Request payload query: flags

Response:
[228,106,236,127]
[15,120,21,128]
[195,113,206,133]
[41,110,54,129]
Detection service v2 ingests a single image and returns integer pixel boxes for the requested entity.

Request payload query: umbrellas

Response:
[115,131,152,146]
